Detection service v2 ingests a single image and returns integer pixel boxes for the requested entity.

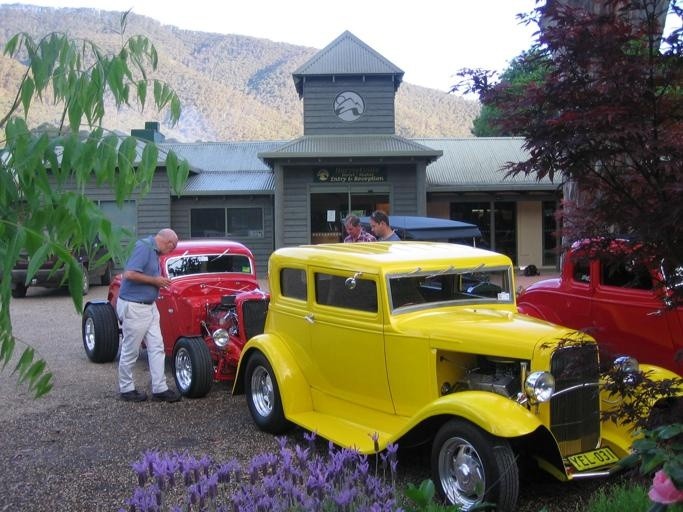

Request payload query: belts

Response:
[118,295,154,305]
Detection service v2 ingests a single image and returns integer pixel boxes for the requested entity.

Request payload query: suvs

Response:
[509,228,682,376]
[7,227,114,300]
[225,237,682,512]
[77,235,271,401]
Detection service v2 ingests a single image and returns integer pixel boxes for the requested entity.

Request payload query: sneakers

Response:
[119,390,148,402]
[151,389,182,403]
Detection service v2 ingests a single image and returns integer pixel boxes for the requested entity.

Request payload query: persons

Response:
[369,210,402,241]
[340,214,377,243]
[113,227,183,404]
